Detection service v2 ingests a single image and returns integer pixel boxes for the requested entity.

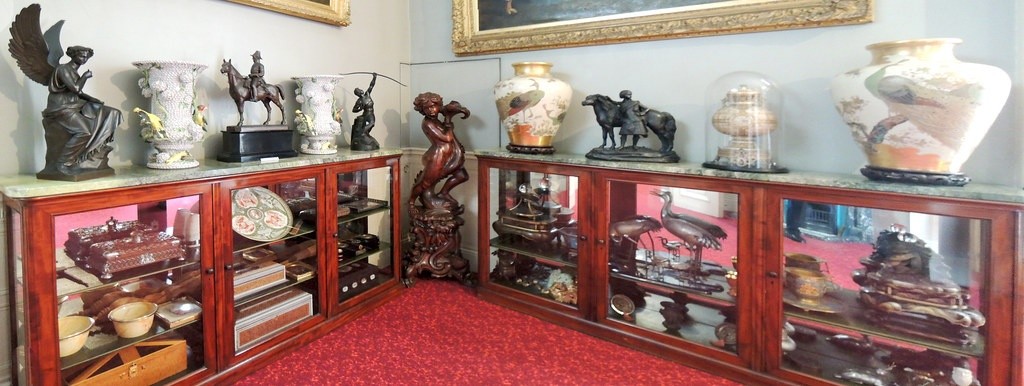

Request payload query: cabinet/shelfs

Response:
[475,145,1024,386]
[0,144,404,386]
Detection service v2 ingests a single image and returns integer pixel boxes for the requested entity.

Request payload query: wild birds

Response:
[608,190,728,282]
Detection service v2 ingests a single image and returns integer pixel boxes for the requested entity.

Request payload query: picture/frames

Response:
[225,0,352,27]
[451,0,874,57]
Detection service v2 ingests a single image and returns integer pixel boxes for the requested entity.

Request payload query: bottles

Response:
[493,62,573,148]
[829,38,1012,174]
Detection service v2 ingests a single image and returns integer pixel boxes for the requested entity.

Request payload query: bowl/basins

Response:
[108,302,158,338]
[58,316,95,357]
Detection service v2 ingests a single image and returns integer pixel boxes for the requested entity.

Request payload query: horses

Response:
[219,58,286,128]
[581,93,677,153]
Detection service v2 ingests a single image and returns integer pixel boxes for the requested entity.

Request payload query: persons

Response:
[407,92,469,206]
[617,89,648,151]
[43,45,124,173]
[352,73,378,136]
[248,51,264,102]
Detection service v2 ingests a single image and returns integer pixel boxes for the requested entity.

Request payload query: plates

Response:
[230,187,293,243]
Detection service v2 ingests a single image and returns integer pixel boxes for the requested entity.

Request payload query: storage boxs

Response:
[63,339,187,386]
[233,261,289,302]
[234,287,313,355]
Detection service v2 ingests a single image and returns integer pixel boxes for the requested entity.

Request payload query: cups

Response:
[183,213,200,247]
[173,208,190,240]
